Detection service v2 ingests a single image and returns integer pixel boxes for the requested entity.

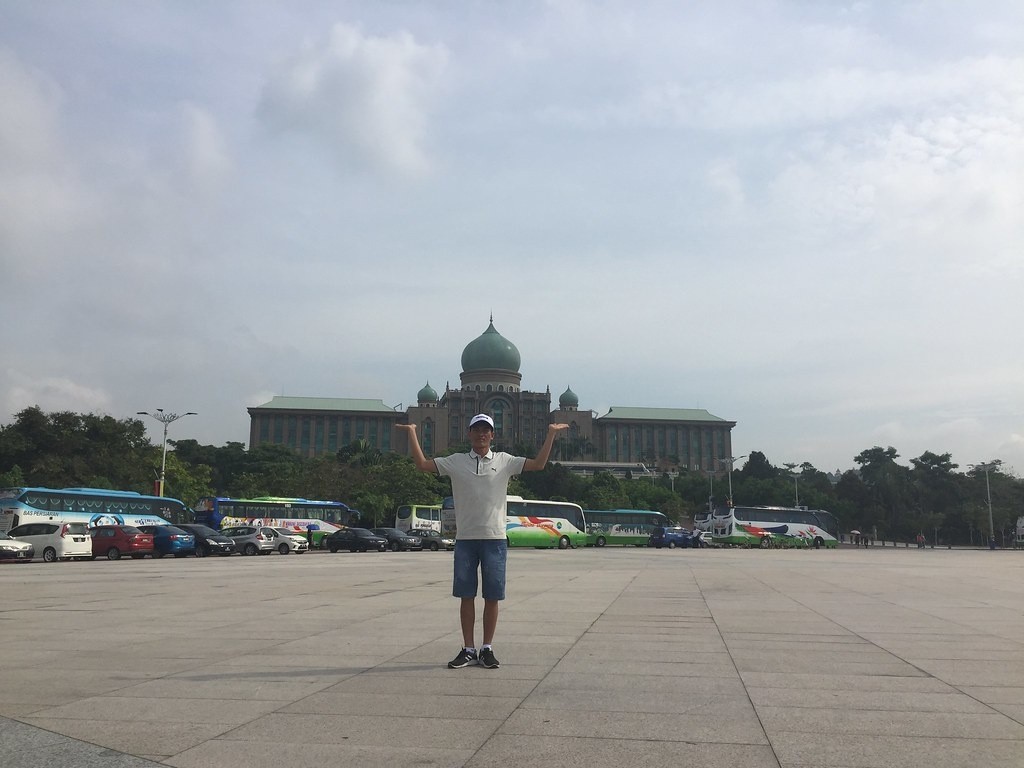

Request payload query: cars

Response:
[0,530,35,563]
[326,528,389,553]
[136,526,195,558]
[406,528,457,551]
[217,526,276,556]
[651,527,732,549]
[266,527,309,555]
[170,524,236,557]
[73,525,154,560]
[369,528,423,552]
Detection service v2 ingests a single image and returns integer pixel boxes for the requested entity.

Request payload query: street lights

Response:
[966,462,1009,549]
[712,456,746,506]
[137,409,198,496]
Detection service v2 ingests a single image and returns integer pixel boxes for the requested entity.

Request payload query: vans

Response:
[6,522,93,563]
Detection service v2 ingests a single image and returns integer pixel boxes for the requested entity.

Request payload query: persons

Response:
[841,533,845,543]
[854,534,860,549]
[917,532,926,548]
[395,413,570,670]
[864,536,868,549]
[850,535,854,544]
[307,529,313,551]
[692,526,701,548]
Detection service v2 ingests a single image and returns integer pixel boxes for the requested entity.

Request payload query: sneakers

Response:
[448,648,477,668]
[478,648,499,667]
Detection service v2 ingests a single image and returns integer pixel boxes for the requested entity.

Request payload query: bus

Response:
[395,504,443,536]
[582,509,669,548]
[0,487,196,534]
[440,495,586,549]
[694,506,841,549]
[193,496,361,549]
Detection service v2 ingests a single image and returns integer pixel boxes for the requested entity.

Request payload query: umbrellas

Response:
[307,524,319,530]
[850,530,861,534]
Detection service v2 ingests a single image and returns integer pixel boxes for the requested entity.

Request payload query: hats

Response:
[469,415,495,432]
